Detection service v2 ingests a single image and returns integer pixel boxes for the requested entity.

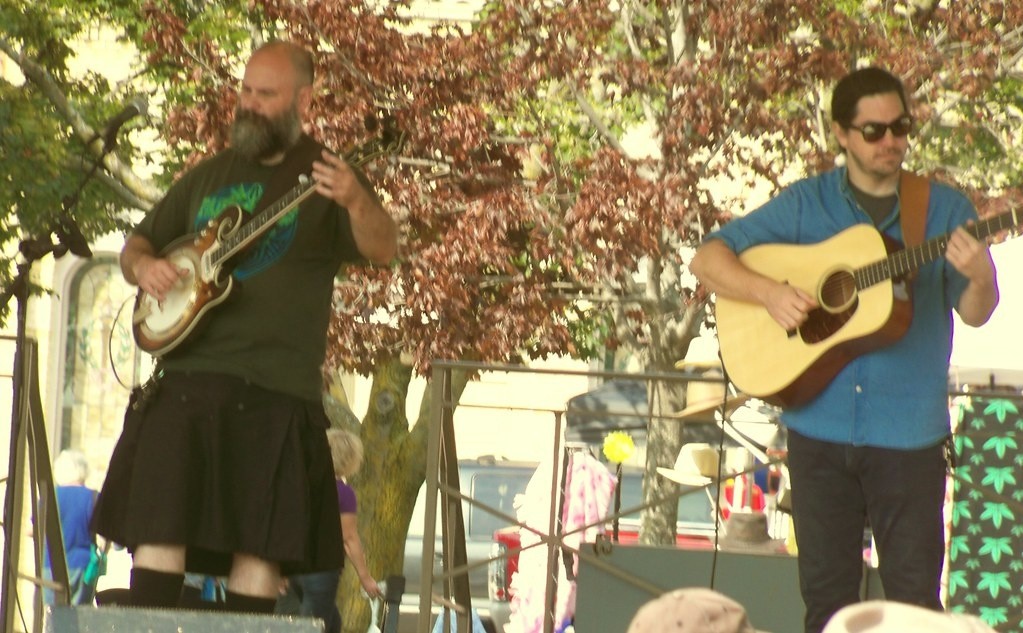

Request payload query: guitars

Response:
[714,203,1023,409]
[131,110,408,359]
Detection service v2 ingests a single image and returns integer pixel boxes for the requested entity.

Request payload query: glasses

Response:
[848,115,913,142]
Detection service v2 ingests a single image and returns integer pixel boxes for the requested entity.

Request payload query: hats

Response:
[627,588,771,633]
[714,513,781,554]
[820,600,1001,633]
[673,371,754,425]
[657,442,721,486]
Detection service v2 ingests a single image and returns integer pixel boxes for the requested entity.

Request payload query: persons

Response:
[278,430,386,632]
[30,450,113,607]
[91,39,398,616]
[689,67,1000,633]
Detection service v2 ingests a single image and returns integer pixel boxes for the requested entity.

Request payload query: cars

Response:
[398,458,538,600]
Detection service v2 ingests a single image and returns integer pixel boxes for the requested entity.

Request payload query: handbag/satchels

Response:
[365,596,382,633]
[82,544,108,588]
